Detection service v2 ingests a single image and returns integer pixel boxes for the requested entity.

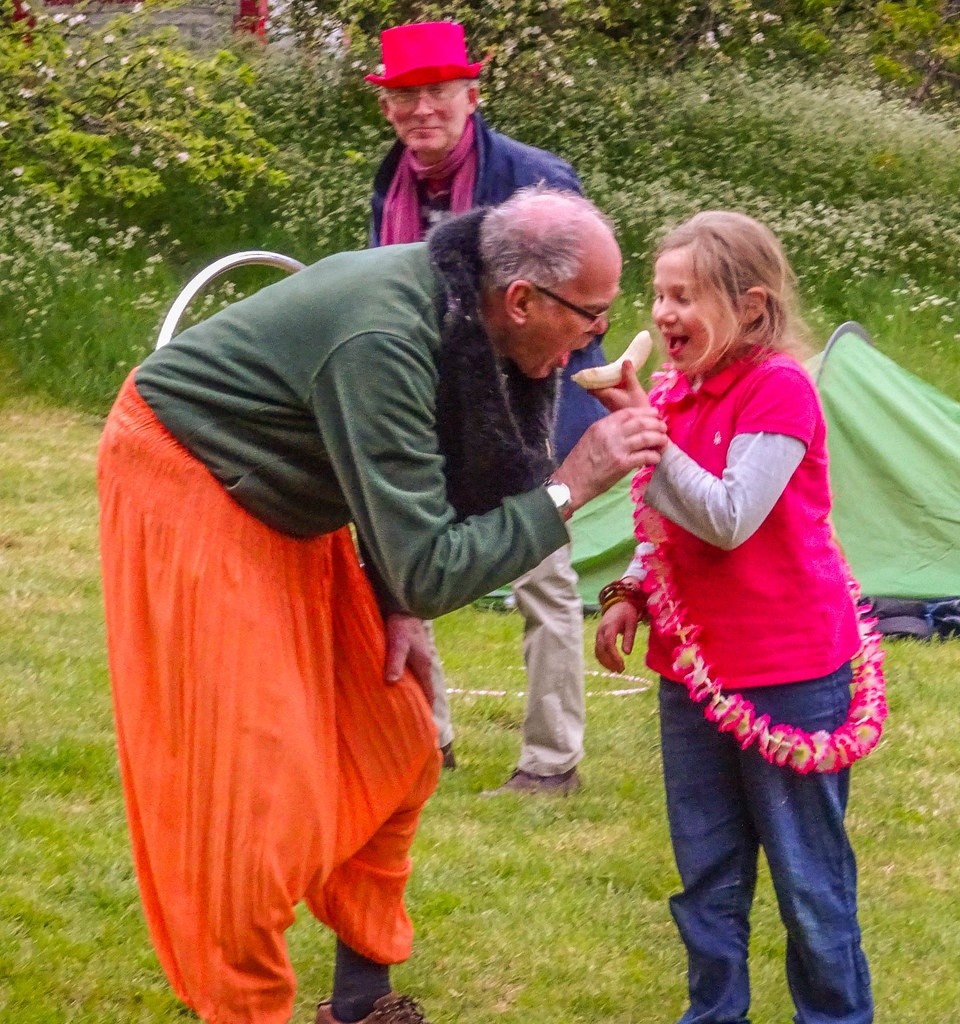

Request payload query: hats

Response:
[364,21,483,88]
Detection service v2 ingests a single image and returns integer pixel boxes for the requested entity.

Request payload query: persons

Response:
[363,21,612,797]
[98,178,666,1024]
[587,209,875,1024]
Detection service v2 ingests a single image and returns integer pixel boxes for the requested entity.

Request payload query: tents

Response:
[473,321,960,621]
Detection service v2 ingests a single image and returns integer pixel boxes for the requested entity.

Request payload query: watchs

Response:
[543,477,572,521]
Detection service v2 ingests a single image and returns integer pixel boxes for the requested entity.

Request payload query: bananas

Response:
[570,329,652,389]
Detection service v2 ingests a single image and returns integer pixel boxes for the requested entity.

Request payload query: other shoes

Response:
[313,986,429,1024]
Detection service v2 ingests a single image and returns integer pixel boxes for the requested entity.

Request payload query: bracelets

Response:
[598,581,644,622]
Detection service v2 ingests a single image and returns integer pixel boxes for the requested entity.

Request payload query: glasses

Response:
[499,278,609,324]
[383,81,476,104]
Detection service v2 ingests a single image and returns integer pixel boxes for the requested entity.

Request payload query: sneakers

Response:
[481,765,583,798]
[438,742,457,769]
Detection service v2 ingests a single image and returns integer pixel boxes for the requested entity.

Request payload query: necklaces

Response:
[631,362,887,774]
